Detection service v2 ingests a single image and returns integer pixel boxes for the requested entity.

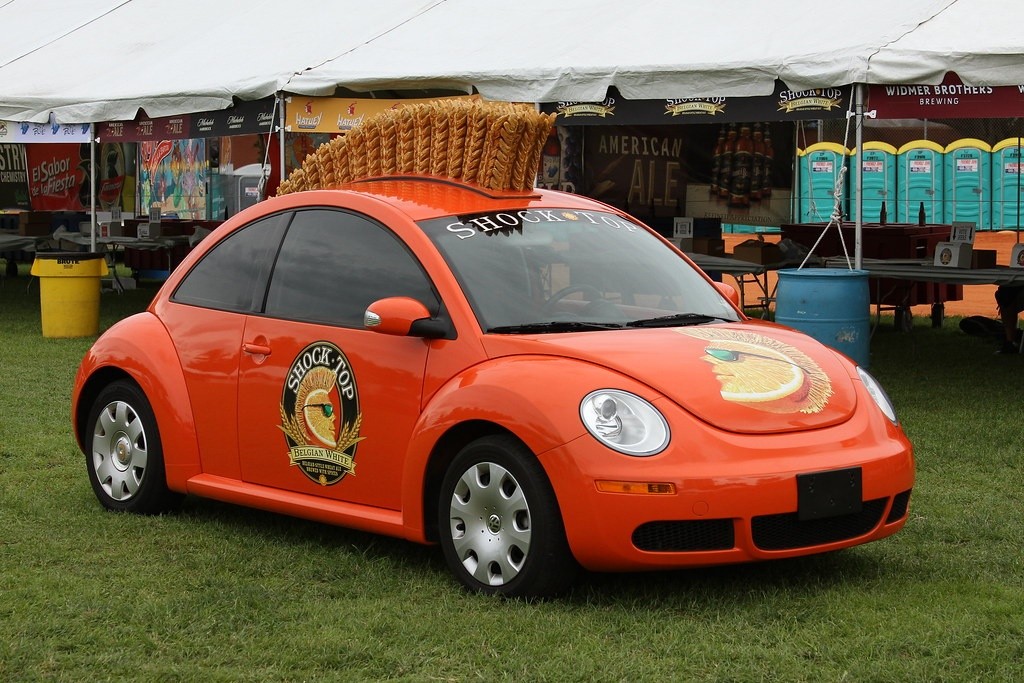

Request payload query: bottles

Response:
[710,123,774,209]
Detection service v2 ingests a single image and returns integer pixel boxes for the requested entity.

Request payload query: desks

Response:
[0,235,52,296]
[686,252,770,321]
[51,230,175,295]
[122,218,223,288]
[821,255,940,312]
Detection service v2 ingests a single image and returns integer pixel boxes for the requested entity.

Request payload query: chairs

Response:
[287,242,348,318]
[78,222,125,290]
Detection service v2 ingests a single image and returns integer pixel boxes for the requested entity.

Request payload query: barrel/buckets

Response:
[773,266,871,375]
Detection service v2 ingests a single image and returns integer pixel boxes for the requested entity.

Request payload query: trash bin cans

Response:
[30,250,110,340]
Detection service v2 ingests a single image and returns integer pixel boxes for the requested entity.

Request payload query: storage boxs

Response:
[112,277,136,290]
[20,211,52,224]
[971,250,996,269]
[734,239,780,265]
[100,222,121,238]
[1010,243,1024,268]
[694,237,725,256]
[19,224,50,236]
[137,222,160,239]
[668,238,693,252]
[934,241,972,270]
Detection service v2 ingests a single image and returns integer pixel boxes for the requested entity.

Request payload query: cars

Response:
[71,174,917,601]
[226,163,271,219]
